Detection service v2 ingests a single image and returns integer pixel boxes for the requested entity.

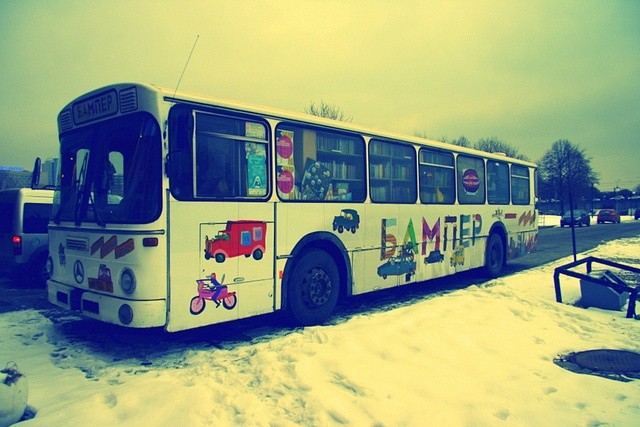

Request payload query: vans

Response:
[1,187,55,281]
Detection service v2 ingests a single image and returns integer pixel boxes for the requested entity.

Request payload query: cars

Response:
[597,208,620,223]
[560,209,591,227]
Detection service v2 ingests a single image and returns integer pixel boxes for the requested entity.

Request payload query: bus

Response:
[46,80,537,327]
